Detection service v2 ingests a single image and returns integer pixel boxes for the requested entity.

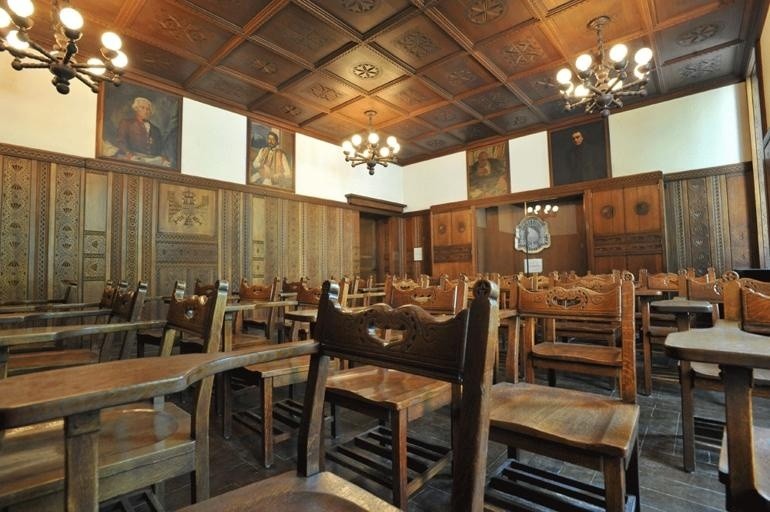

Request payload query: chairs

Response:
[0,268,770,511]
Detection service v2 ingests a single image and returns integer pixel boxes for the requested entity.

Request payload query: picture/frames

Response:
[547,118,611,188]
[96,77,183,173]
[246,116,295,195]
[466,140,511,200]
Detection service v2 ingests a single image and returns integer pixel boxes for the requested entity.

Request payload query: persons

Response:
[565,130,597,164]
[470,150,505,199]
[114,97,170,167]
[249,131,293,188]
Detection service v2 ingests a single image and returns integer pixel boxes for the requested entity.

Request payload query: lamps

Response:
[0,0,129,96]
[526,204,559,218]
[342,109,401,172]
[555,15,654,119]
